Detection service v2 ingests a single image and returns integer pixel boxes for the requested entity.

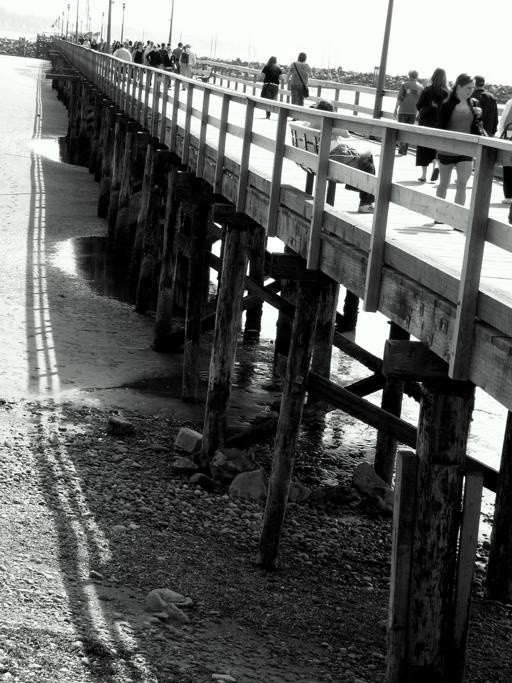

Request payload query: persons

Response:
[491,97,512,225]
[467,73,499,137]
[393,68,425,156]
[260,55,283,118]
[308,98,377,213]
[284,51,313,121]
[414,67,452,182]
[54,34,214,90]
[434,72,487,232]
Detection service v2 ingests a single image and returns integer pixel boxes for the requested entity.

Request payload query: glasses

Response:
[138,44,142,45]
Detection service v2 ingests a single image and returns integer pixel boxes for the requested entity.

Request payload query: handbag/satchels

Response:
[181,52,189,63]
[303,86,309,98]
[329,143,361,167]
[472,119,489,137]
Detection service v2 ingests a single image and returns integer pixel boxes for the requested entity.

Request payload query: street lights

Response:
[50,0,180,78]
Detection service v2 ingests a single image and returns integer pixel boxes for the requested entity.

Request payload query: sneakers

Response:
[399,150,407,155]
[359,204,376,213]
[169,84,186,90]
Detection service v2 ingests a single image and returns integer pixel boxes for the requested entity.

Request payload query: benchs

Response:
[289,122,360,206]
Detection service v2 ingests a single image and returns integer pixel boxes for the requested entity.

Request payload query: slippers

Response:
[417,168,440,182]
